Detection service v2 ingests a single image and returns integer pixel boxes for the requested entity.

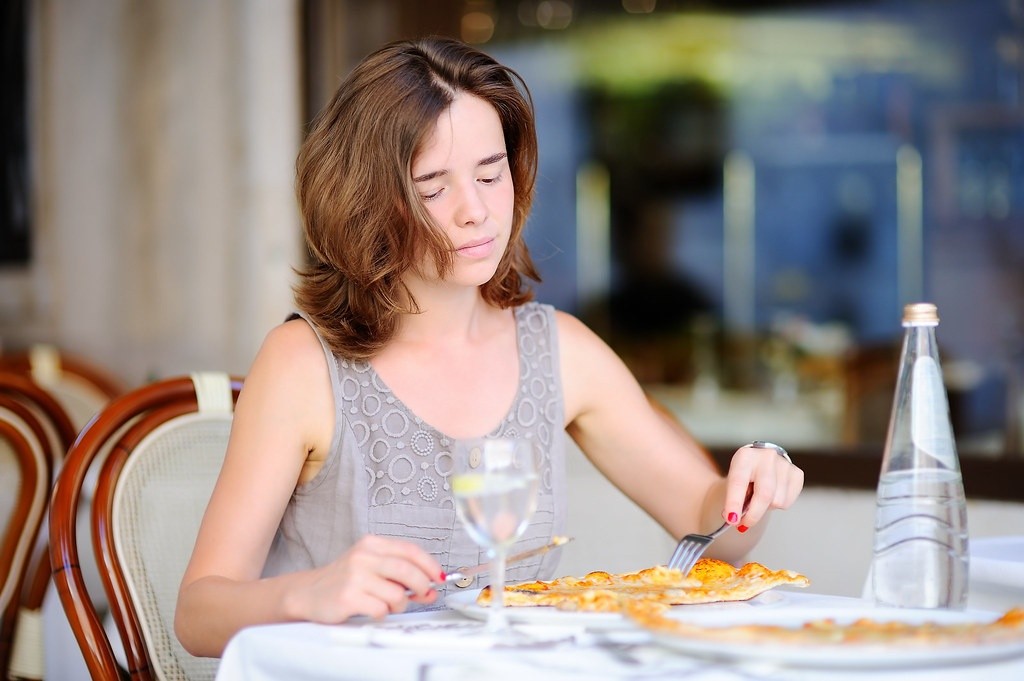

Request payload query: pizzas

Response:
[635,604,1023,648]
[476,560,808,610]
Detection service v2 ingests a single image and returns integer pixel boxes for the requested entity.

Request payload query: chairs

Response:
[0,349,247,681]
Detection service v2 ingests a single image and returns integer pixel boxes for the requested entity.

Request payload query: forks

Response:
[668,492,752,577]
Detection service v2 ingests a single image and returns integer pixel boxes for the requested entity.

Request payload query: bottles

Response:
[872,303,970,606]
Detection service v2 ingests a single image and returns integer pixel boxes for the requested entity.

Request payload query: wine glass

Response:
[448,436,539,645]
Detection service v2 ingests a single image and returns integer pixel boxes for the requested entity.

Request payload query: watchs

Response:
[737,440,792,462]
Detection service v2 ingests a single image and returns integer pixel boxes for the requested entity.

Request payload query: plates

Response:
[446,562,808,618]
[648,607,1024,664]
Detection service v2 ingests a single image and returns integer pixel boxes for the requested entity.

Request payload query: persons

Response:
[173,33,804,658]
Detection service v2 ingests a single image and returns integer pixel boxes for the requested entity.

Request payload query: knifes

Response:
[404,537,575,598]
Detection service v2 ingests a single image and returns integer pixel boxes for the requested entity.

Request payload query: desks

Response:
[216,587,1024,681]
